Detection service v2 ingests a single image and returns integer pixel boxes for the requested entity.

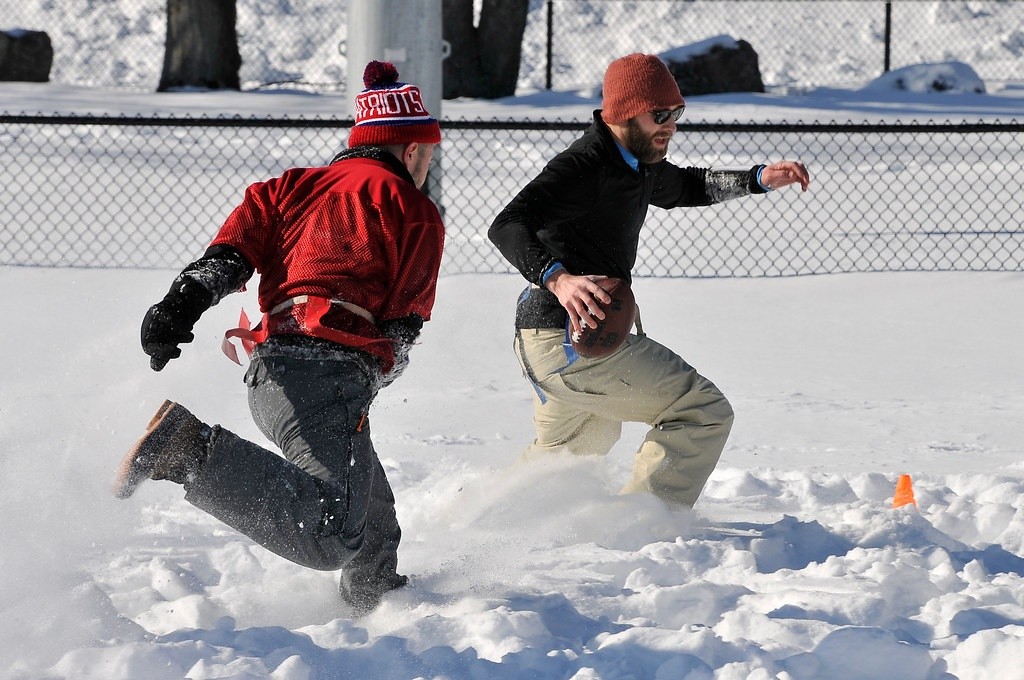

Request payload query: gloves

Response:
[378,350,408,386]
[140,274,214,372]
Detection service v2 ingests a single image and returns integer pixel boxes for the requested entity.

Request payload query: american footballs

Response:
[567,277,636,359]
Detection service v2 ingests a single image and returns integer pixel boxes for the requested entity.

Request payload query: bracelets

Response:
[539,256,563,286]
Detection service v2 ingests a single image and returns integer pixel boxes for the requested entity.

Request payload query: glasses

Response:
[648,106,685,124]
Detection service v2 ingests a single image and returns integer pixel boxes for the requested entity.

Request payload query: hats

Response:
[602,53,685,123]
[348,60,441,148]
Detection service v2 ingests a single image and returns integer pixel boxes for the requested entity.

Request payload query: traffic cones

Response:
[889,475,917,510]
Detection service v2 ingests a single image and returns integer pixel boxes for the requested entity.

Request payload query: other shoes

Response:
[114,399,207,498]
[387,573,408,586]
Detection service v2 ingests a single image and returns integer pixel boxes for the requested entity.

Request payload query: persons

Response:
[486,54,809,508]
[114,59,446,614]
